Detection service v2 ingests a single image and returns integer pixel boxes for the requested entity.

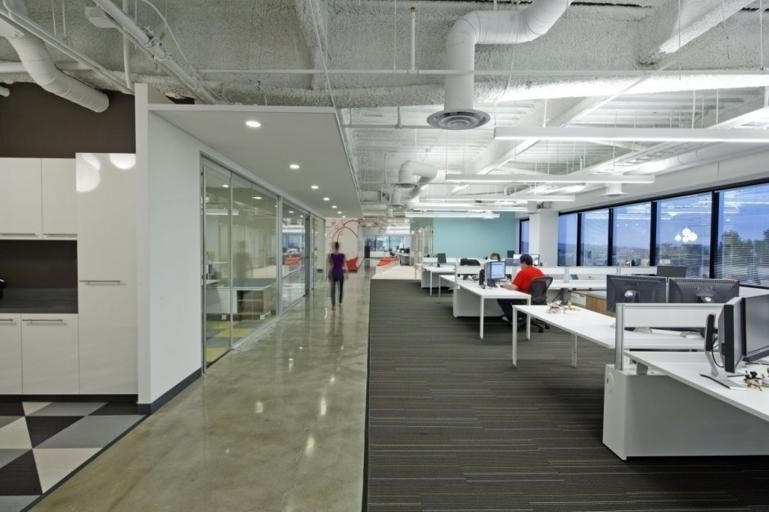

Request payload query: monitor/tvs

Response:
[461,259,480,266]
[700,296,749,389]
[529,254,541,266]
[606,274,667,314]
[437,253,446,264]
[513,254,523,260]
[657,266,687,277]
[484,261,506,289]
[0,239,79,315]
[742,294,769,365]
[667,277,739,303]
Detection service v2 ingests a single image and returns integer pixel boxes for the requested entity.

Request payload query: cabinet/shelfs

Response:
[1,79,137,413]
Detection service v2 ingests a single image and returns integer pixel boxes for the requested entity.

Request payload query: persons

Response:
[489,252,501,261]
[326,242,349,311]
[232,239,254,280]
[328,304,344,352]
[496,254,548,324]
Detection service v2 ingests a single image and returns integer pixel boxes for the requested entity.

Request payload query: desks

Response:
[415,257,769,461]
